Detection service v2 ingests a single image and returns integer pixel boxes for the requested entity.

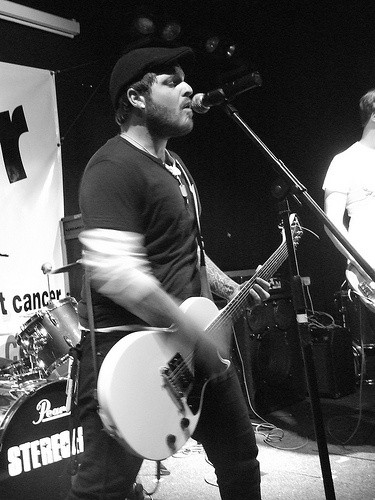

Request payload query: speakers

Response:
[309,327,357,399]
[215,291,307,419]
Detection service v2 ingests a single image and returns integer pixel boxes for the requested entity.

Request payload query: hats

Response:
[108,46,196,114]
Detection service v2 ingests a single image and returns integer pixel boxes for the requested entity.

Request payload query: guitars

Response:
[344,259,375,313]
[95,210,305,463]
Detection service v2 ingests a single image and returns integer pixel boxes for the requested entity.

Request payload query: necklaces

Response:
[122,133,189,206]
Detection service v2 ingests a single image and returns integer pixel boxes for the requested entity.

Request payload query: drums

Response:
[13,295,84,384]
[0,357,48,394]
[0,378,145,500]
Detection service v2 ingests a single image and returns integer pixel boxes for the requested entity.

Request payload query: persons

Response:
[318,93,375,415]
[77,45,263,500]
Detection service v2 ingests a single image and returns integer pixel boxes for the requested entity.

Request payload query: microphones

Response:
[191,71,264,113]
